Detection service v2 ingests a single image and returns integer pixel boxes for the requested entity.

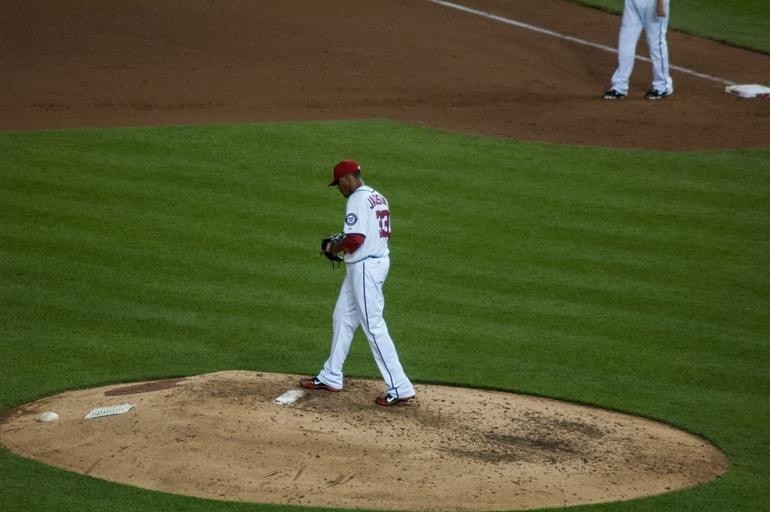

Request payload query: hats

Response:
[328,160,361,186]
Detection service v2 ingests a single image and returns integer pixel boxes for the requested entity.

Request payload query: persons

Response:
[297,159,417,407]
[603,0,674,100]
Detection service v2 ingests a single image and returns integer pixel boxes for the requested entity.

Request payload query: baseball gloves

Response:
[320,234,343,263]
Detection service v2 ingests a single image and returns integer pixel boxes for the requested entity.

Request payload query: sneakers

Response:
[604,89,625,100]
[300,376,342,392]
[644,88,674,100]
[376,393,416,406]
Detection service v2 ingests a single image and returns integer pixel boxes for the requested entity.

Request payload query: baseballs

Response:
[39,412,58,422]
